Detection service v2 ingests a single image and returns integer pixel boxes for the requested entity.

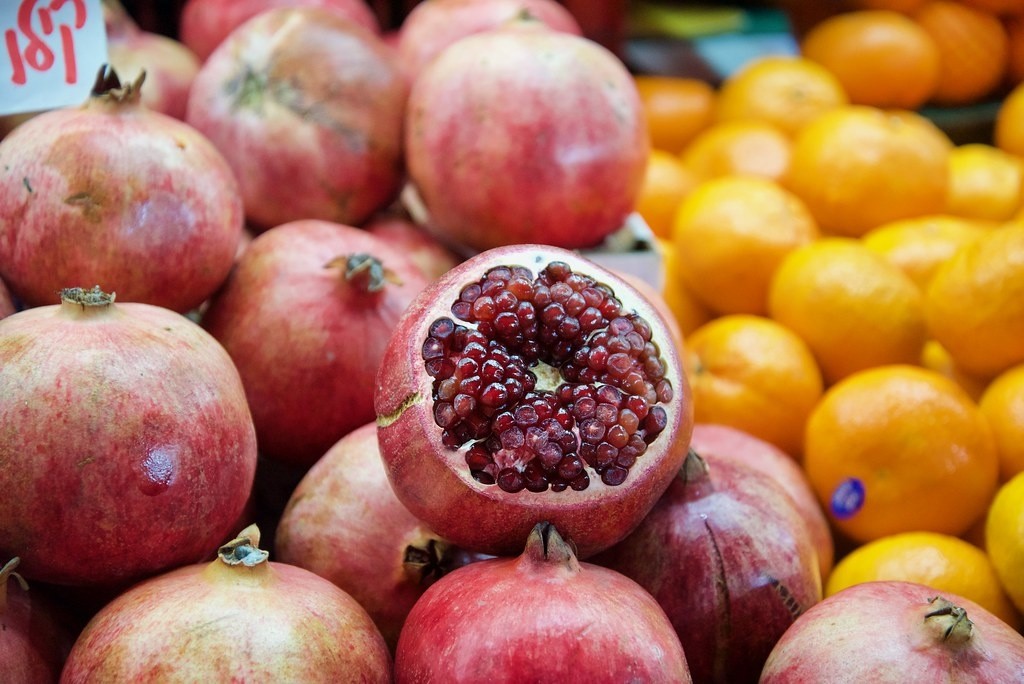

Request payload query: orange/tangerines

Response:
[628,0,1024,626]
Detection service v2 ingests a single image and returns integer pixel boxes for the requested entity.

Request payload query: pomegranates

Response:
[1,0,832,684]
[763,579,1024,684]
